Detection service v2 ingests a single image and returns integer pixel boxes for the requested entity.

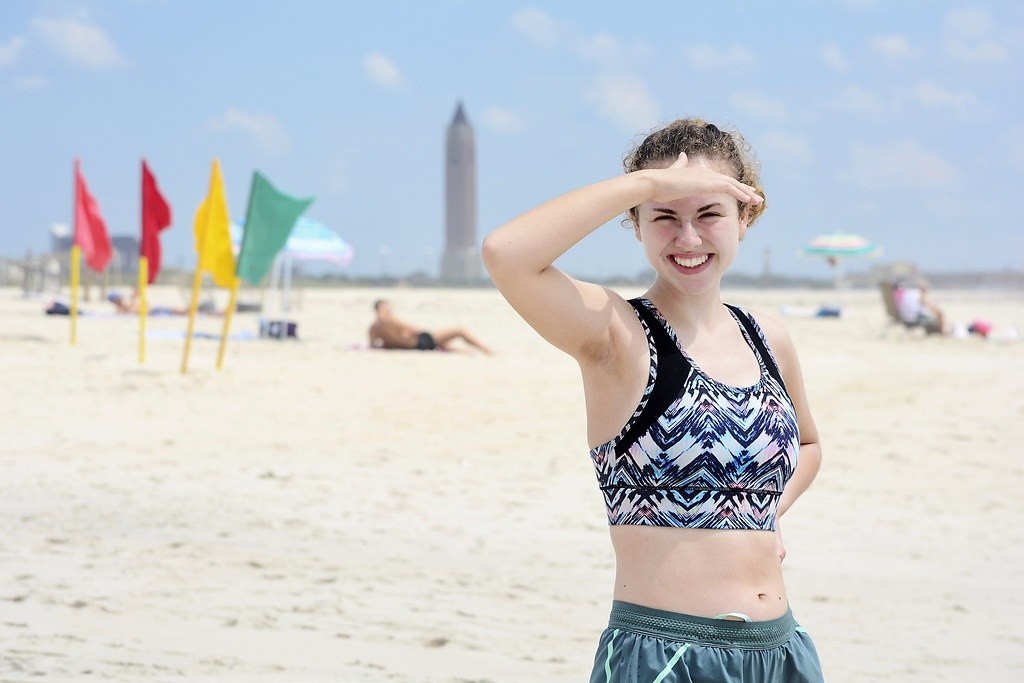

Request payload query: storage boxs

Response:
[262,321,296,338]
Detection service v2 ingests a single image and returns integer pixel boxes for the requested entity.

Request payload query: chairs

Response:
[882,282,943,338]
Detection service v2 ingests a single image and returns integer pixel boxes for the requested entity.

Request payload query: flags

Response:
[181,163,241,291]
[220,170,315,289]
[65,159,111,275]
[136,160,170,285]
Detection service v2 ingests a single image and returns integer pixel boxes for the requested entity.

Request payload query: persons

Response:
[368,301,496,357]
[483,120,826,682]
[893,276,941,334]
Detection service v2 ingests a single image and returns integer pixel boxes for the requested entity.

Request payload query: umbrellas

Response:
[228,216,352,323]
[805,232,879,314]
